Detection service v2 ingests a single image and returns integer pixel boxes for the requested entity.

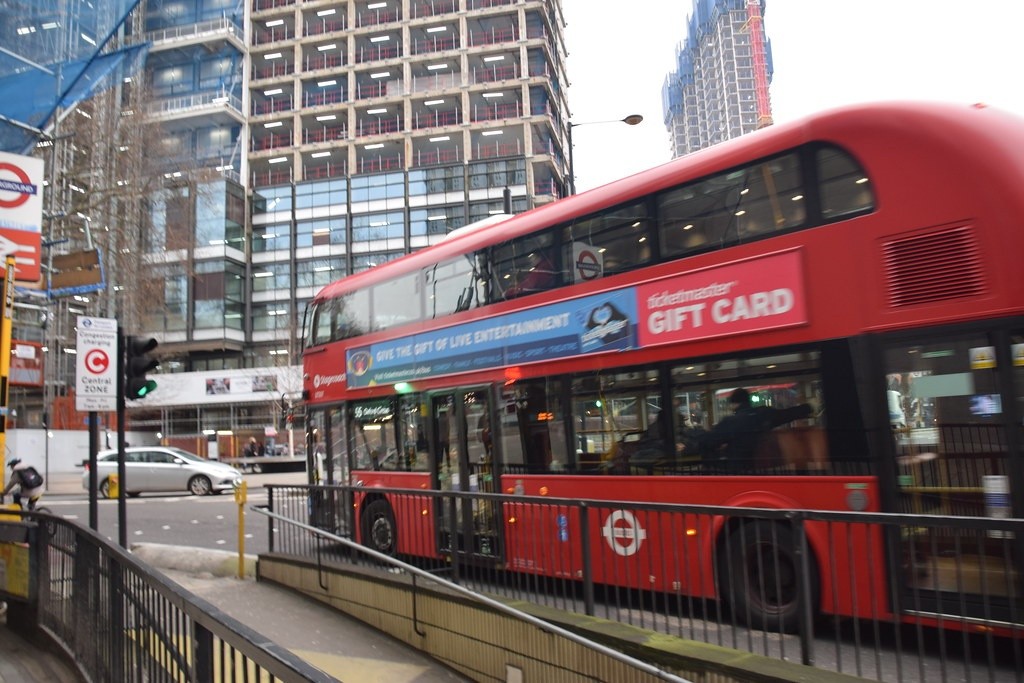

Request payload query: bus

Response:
[277,96,1024,640]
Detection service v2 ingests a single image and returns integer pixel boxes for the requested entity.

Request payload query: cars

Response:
[80,445,243,498]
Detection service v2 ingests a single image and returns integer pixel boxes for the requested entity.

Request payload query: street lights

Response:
[567,114,643,194]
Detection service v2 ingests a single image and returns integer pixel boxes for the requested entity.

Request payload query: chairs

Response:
[619,428,646,475]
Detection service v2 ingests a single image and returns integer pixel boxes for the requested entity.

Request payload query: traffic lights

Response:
[125,335,159,398]
[750,395,761,402]
[589,400,602,409]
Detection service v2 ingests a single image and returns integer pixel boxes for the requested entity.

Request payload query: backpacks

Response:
[18,466,43,490]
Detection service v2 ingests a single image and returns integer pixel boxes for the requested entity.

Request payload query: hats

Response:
[6,459,23,466]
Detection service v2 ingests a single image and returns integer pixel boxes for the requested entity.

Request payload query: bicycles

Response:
[1,491,57,540]
[236,452,264,474]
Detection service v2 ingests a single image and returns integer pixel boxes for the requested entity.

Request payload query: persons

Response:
[481,421,493,458]
[3,458,44,510]
[246,437,264,456]
[676,387,819,475]
[500,249,550,299]
[438,411,452,469]
